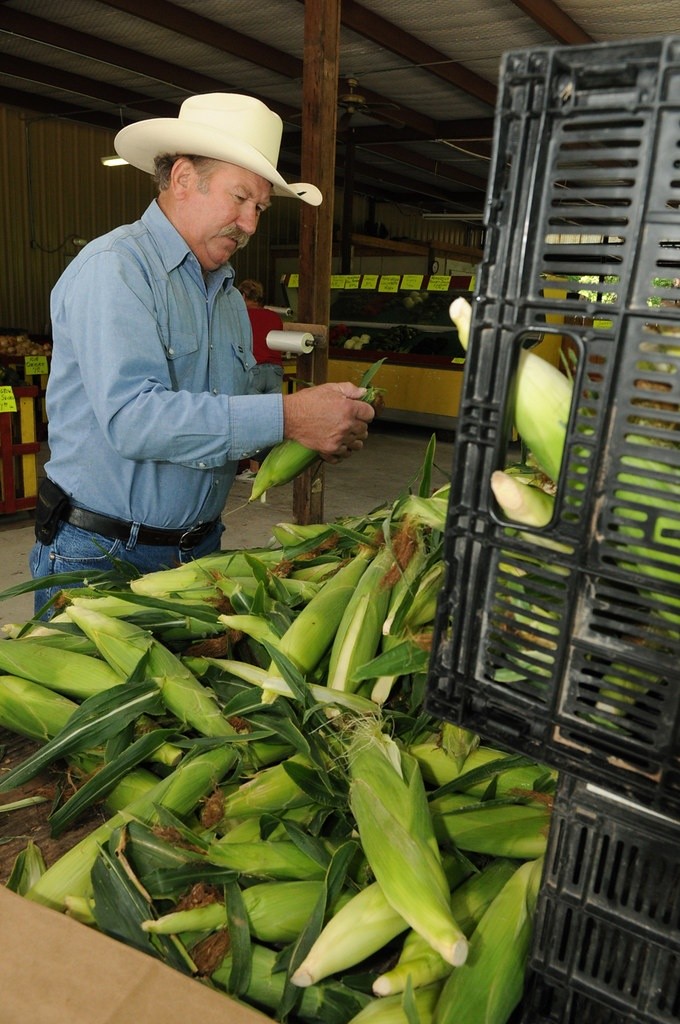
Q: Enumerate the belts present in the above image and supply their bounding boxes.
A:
[60,505,220,551]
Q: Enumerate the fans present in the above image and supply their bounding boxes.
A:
[288,67,406,131]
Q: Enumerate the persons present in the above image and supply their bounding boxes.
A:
[31,93,375,623]
[234,280,284,481]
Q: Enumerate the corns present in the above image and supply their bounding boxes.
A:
[0,296,680,1024]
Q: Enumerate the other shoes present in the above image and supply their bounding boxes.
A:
[235,468,258,483]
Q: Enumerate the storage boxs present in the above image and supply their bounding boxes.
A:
[424,37,680,1024]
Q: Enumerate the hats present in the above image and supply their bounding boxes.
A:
[114,92,325,206]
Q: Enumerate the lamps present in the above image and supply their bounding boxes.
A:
[100,108,132,168]
[421,213,484,221]
